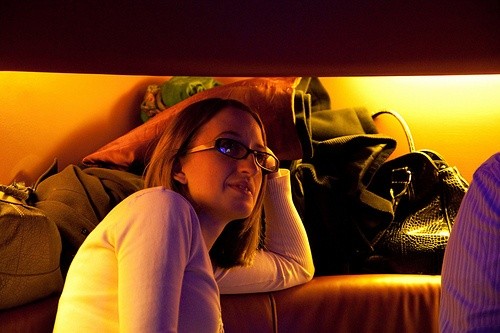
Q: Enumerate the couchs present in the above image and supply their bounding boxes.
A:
[0,273,442,333]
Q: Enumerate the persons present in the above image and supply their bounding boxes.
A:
[56,95,315,333]
[436,149,500,333]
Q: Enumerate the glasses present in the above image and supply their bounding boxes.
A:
[183,137,280,174]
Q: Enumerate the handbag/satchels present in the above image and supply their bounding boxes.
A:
[370,110,471,271]
